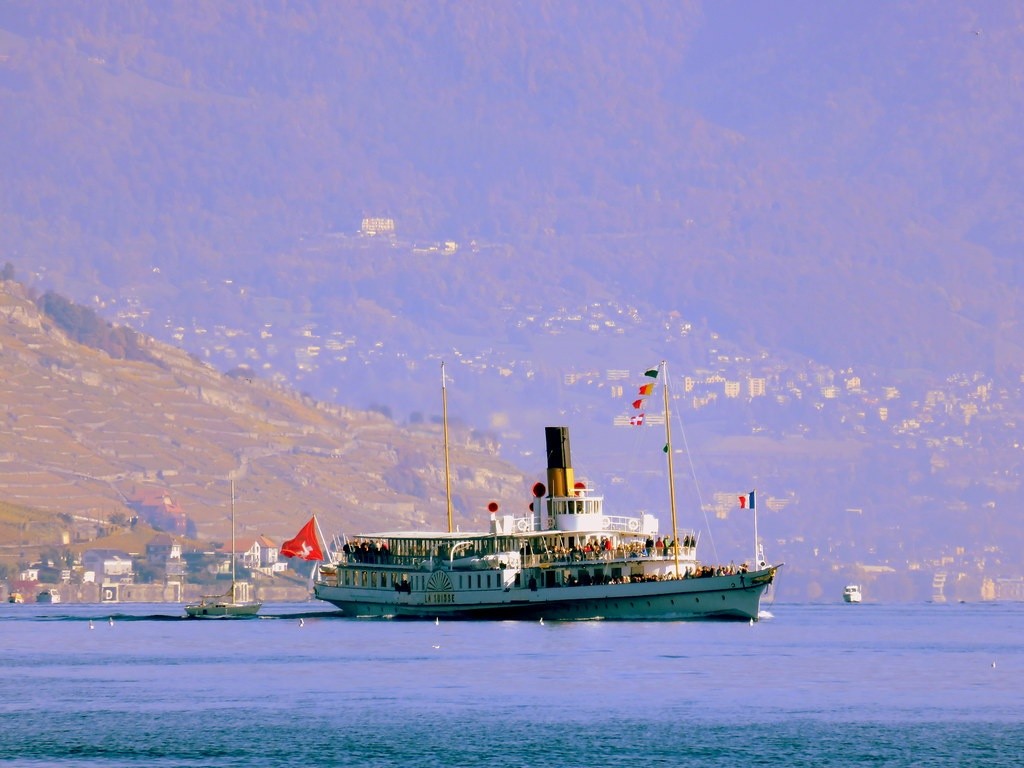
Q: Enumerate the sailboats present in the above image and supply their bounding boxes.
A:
[184,479,262,619]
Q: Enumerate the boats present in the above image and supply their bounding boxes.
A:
[8,593,22,603]
[313,359,785,622]
[843,585,862,602]
[36,589,60,604]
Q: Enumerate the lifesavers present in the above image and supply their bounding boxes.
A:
[602,517,610,528]
[517,520,527,531]
[628,519,638,531]
[548,517,555,528]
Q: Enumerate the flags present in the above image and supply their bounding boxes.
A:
[280,518,324,561]
[639,383,654,396]
[645,365,659,378]
[739,491,754,509]
[630,413,644,425]
[632,399,646,410]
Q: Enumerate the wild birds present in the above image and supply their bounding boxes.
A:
[432,645,440,648]
[991,661,996,668]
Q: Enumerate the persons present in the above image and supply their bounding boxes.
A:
[566,564,750,590]
[343,538,389,564]
[546,535,695,565]
[577,504,582,513]
